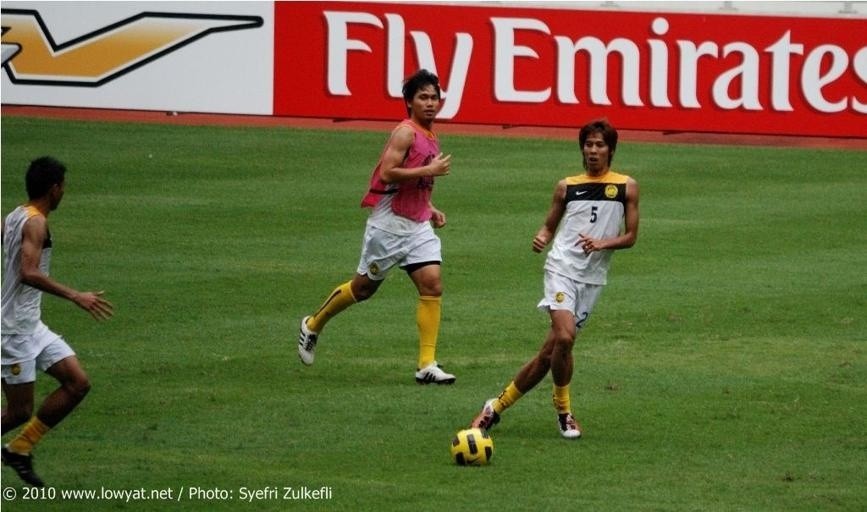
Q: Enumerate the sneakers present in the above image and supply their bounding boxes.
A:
[298,315,320,367]
[471,397,500,431]
[414,360,458,385]
[2,444,46,489]
[557,411,581,439]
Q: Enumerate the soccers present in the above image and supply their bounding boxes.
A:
[450,428,494,466]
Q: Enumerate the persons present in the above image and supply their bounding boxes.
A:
[0,155,115,489]
[296,68,458,386]
[468,118,640,439]
